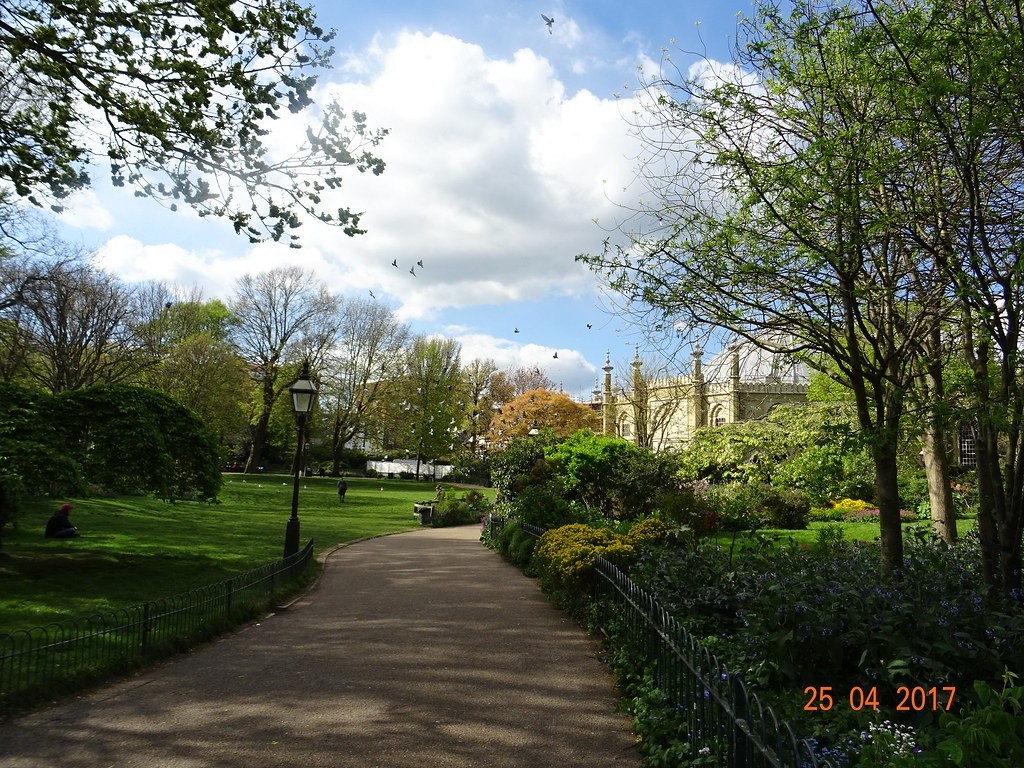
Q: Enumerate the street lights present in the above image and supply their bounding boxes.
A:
[280,355,318,561]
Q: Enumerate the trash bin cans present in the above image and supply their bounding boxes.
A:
[418,509,431,525]
[306,468,312,476]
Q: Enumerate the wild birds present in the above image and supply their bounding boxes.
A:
[541,14,554,34]
[410,266,415,276]
[515,328,519,333]
[397,352,559,461]
[587,323,592,329]
[225,453,389,492]
[418,260,423,268]
[369,290,375,298]
[553,352,557,358]
[392,259,397,267]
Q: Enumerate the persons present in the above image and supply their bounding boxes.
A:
[317,468,325,478]
[308,467,312,476]
[337,478,347,503]
[231,461,245,472]
[45,504,80,539]
[435,485,445,500]
